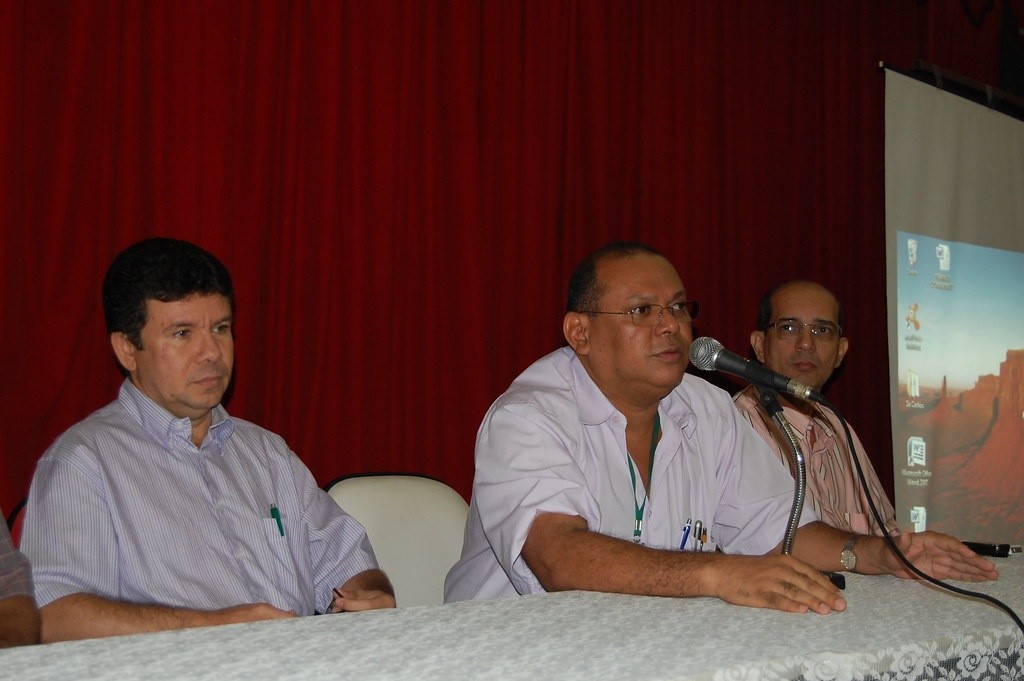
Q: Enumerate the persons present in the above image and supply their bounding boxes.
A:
[0,509,40,649]
[730,280,901,536]
[20,236,398,644]
[443,241,1000,615]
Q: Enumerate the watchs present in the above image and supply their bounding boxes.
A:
[841,534,863,573]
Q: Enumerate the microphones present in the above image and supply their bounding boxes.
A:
[689,336,833,409]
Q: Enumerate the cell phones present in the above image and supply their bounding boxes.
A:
[958,540,1010,559]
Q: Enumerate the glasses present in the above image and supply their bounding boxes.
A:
[760,315,842,340]
[575,300,700,326]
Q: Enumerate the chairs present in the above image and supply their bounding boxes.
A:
[328,471,471,608]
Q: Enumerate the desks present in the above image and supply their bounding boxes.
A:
[1,547,1024,680]
